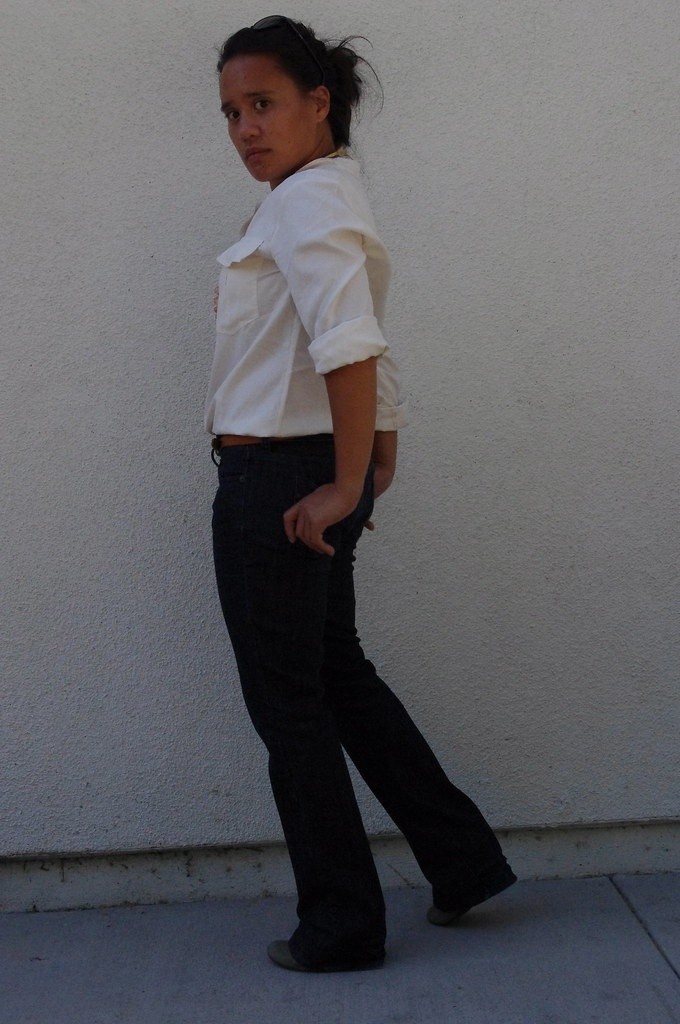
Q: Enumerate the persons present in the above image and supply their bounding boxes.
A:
[200,13,519,973]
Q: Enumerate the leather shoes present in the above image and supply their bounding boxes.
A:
[425,875,516,926]
[267,939,303,971]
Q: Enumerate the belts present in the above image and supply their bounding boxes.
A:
[215,435,263,450]
